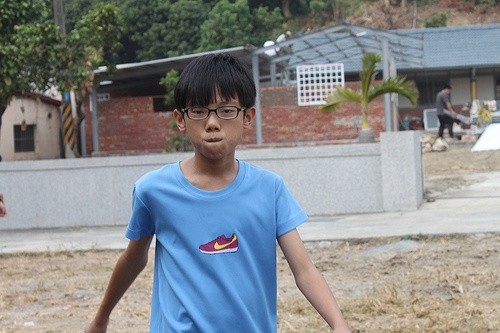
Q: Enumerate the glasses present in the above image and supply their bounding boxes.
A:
[181,106,247,121]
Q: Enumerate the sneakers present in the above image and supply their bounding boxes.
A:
[199,233,238,253]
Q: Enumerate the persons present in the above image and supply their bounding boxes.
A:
[275,30,292,56]
[436,84,458,140]
[84,53,352,333]
